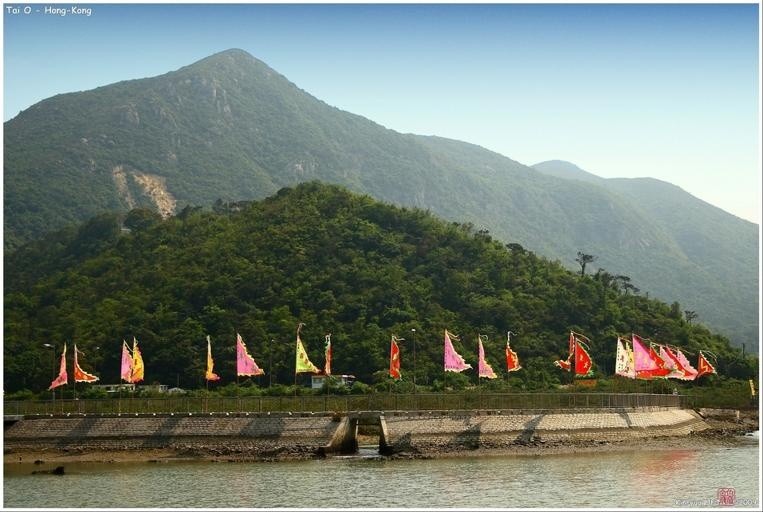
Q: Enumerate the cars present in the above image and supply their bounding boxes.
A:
[166,387,186,394]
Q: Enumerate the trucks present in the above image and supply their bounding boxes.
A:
[310,374,358,389]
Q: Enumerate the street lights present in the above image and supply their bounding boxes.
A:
[42,342,56,414]
[411,327,418,394]
[93,346,100,374]
